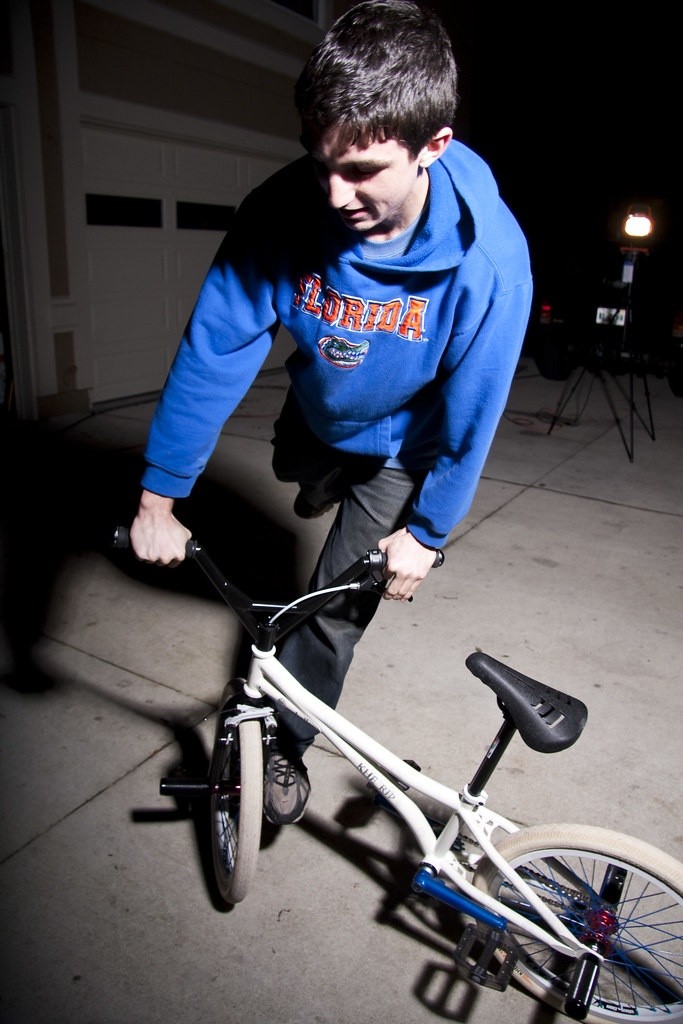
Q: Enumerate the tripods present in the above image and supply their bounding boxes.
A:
[547,247,656,464]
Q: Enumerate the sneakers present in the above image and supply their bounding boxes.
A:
[262,735,312,826]
[292,486,337,520]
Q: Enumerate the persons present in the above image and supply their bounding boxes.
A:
[129,0,533,826]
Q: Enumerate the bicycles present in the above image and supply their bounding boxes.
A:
[108,525,683,1024]
